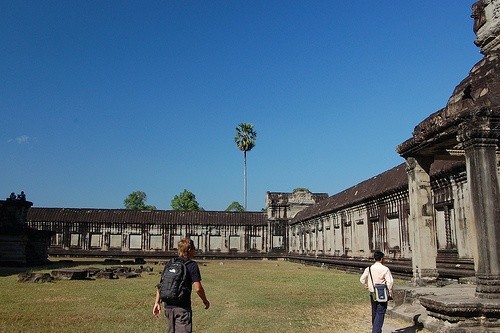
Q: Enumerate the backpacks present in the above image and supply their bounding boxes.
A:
[154,258,194,304]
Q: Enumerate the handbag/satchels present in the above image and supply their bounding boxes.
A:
[372,284,389,302]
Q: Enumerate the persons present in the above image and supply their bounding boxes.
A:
[359,250,394,332]
[152,237,210,333]
